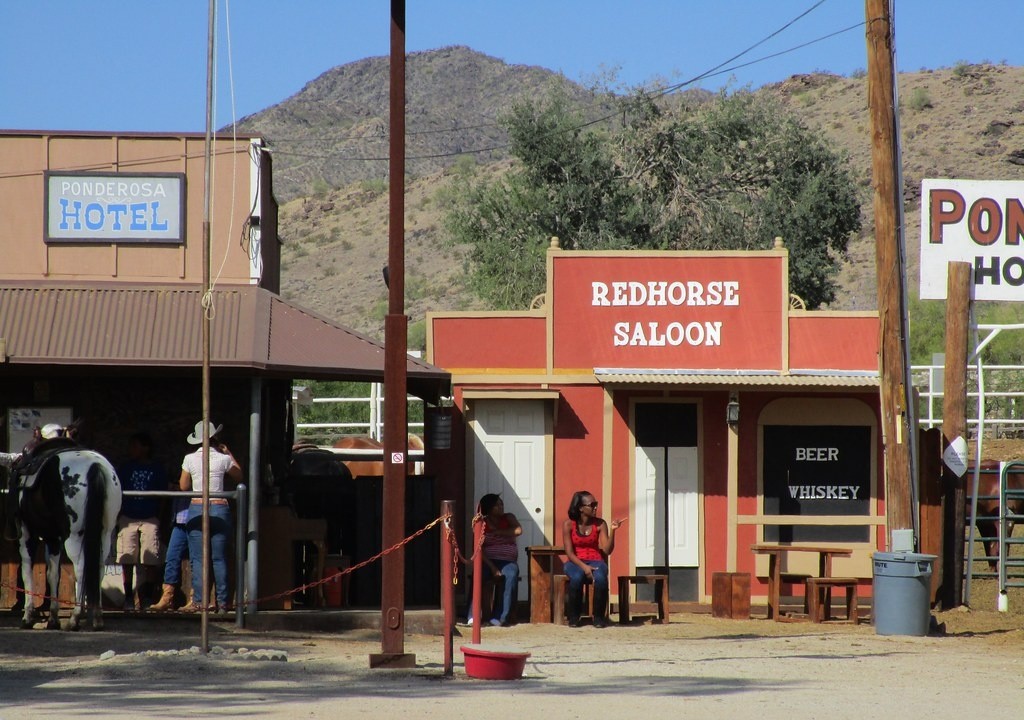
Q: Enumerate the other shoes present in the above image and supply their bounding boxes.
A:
[122,600,135,610]
[489,618,501,626]
[467,618,473,626]
[568,616,581,627]
[593,620,604,627]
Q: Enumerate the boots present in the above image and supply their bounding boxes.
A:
[150,583,175,612]
[178,589,202,613]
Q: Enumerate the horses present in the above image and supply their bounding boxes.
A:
[965,459,1024,577]
[291,431,424,480]
[0,418,124,631]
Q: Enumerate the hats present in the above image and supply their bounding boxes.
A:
[187,420,223,445]
[480,492,502,516]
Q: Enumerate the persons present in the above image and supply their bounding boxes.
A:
[563,491,628,628]
[467,494,523,626]
[150,431,201,613]
[178,420,243,613]
[117,436,174,612]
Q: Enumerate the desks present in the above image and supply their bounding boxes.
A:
[181,505,327,610]
[524,545,608,624]
[748,543,853,623]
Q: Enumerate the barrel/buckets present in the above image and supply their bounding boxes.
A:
[315,567,341,605]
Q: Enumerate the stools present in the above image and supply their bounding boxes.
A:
[807,576,858,625]
[553,575,610,625]
[617,575,669,626]
[466,574,522,622]
[711,571,752,619]
[779,573,812,584]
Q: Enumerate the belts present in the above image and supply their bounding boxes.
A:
[191,499,228,505]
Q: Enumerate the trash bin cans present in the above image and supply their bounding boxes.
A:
[870,552,938,636]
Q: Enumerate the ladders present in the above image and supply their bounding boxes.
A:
[1000,461,1024,591]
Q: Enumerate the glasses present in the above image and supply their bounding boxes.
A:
[584,501,598,507]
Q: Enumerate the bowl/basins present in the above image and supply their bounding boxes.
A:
[462,646,531,679]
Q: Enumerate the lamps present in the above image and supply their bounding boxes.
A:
[429,413,453,455]
[726,394,740,427]
